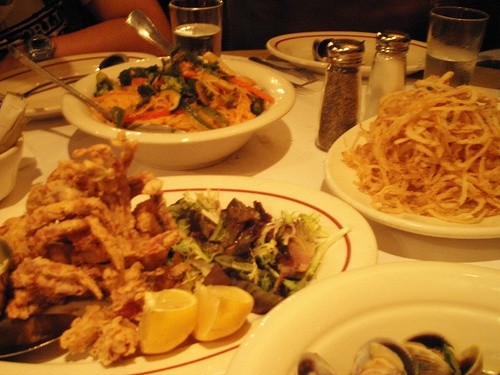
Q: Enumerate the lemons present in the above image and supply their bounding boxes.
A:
[138,288,199,354]
[190,284,254,342]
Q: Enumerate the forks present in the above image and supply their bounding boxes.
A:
[249,56,316,80]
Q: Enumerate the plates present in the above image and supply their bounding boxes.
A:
[0,174,377,375]
[225,260,500,375]
[324,84,500,239]
[266,31,428,80]
[0,52,158,121]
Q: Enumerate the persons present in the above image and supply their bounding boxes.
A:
[0,0,173,73]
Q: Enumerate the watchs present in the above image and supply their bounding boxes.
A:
[27,33,56,62]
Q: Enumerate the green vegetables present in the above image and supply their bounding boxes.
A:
[164,190,350,314]
[92,47,263,132]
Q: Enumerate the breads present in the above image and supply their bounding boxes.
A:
[0,93,27,154]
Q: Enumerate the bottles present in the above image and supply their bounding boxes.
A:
[315,38,365,152]
[364,31,411,119]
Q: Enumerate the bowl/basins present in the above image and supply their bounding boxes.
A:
[60,55,297,171]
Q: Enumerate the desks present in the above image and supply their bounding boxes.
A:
[0,50,500,375]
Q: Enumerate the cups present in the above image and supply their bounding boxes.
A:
[423,5,489,87]
[168,0,223,57]
